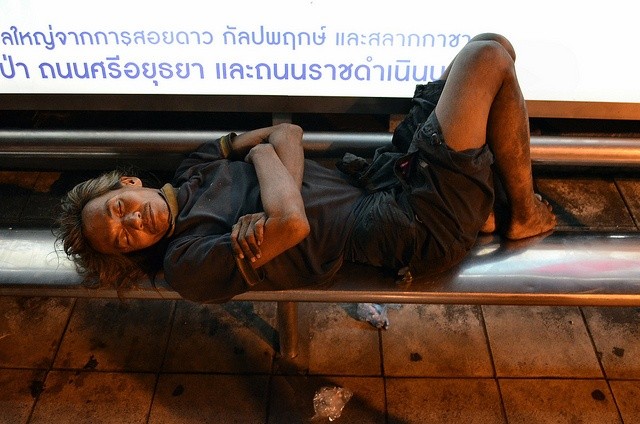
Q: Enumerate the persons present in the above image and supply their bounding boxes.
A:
[52,33,558,303]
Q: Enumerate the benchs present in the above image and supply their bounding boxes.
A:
[1,115,640,309]
